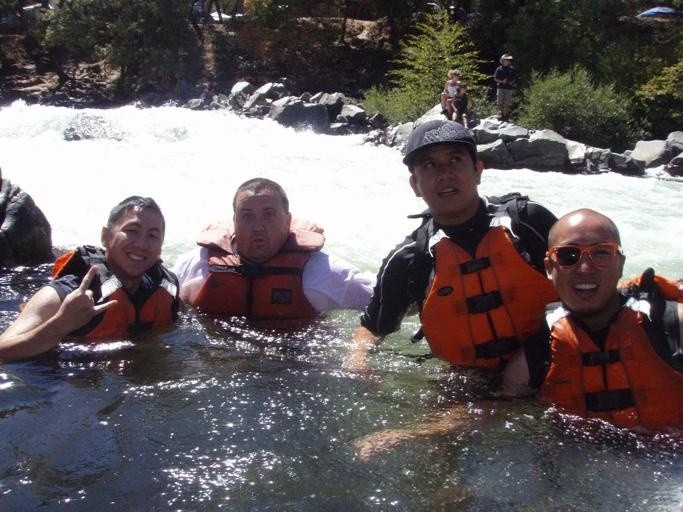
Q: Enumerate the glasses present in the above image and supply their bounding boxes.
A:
[546,244,626,268]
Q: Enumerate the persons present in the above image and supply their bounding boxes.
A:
[180,178,377,328]
[351,208,683,463]
[0,195,178,365]
[341,119,558,376]
[441,52,517,130]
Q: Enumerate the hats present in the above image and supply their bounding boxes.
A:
[403,119,477,168]
